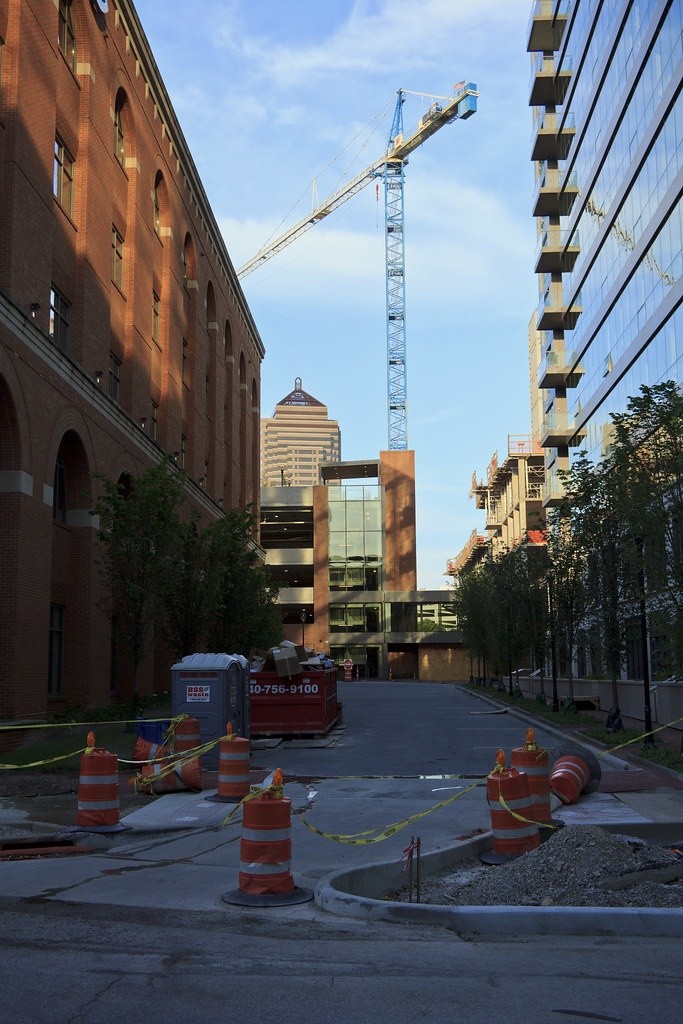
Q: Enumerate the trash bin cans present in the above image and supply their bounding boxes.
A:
[136,717,170,746]
[250,667,339,736]
[170,653,251,771]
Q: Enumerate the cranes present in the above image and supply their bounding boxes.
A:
[237,81,479,451]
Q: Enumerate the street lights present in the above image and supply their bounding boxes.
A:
[633,523,657,749]
[545,559,557,714]
[300,609,306,648]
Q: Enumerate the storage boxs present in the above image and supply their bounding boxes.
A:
[135,717,170,746]
[249,637,339,736]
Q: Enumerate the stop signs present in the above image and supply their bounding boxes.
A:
[344,659,353,670]
